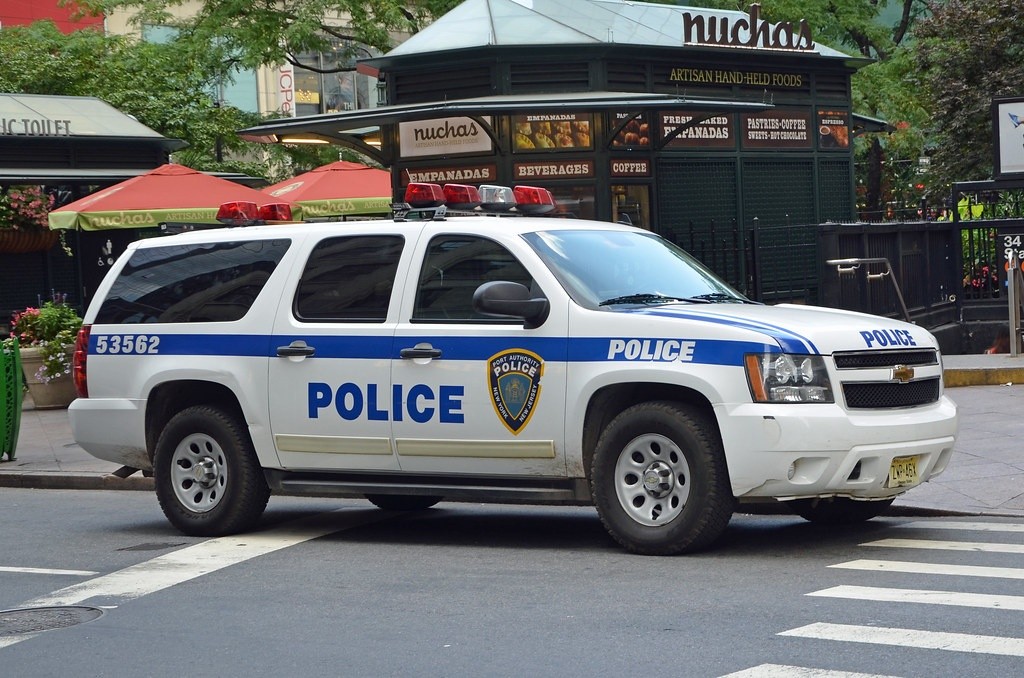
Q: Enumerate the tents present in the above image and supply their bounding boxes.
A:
[48,160,304,317]
[260,161,393,222]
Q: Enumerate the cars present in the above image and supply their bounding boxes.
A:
[67,180,960,556]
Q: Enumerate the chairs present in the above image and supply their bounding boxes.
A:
[444,268,485,320]
[413,267,449,320]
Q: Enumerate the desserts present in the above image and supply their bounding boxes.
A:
[517,121,589,149]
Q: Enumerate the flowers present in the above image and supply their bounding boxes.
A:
[8,288,83,386]
[0,185,73,258]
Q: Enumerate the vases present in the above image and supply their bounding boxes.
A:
[0,226,61,254]
[16,343,83,410]
